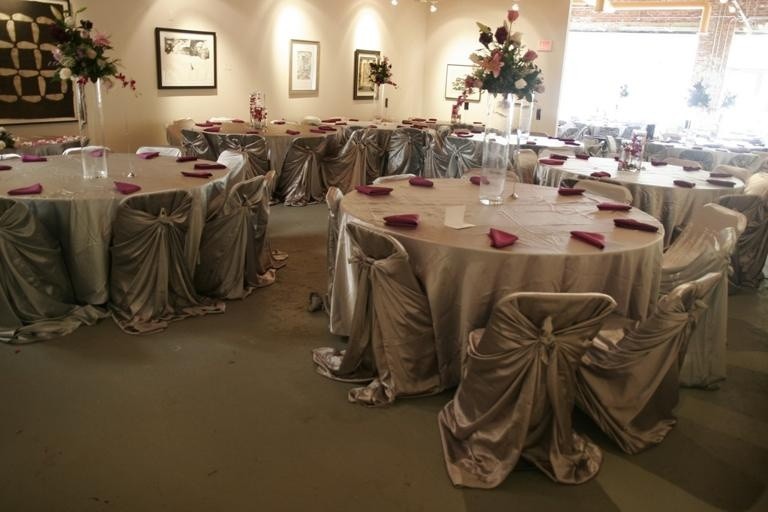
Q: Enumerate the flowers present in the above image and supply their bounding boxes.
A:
[42,4,145,124]
[368,56,398,100]
[451,10,545,120]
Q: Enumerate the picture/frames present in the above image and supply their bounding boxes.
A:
[155,27,217,89]
[354,49,380,101]
[289,38,320,98]
[445,64,481,103]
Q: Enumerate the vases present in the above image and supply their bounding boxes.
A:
[478,92,514,206]
[517,91,535,140]
[373,83,385,125]
[74,76,109,180]
